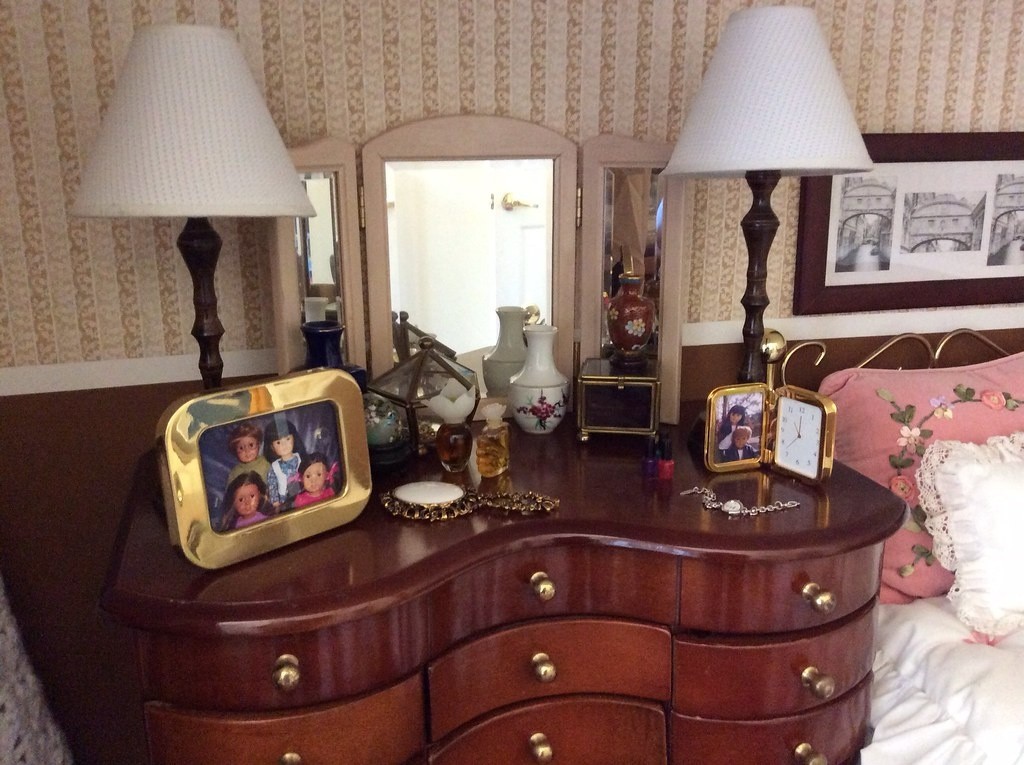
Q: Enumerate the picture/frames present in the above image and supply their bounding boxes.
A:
[150,363,375,574]
[791,130,1024,316]
[703,382,771,474]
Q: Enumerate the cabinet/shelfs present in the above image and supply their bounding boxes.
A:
[99,422,914,764]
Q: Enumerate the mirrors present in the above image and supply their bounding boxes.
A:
[580,133,687,358]
[271,136,368,384]
[359,114,579,424]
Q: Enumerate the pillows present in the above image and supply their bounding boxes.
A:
[917,431,1023,641]
[814,351,1024,607]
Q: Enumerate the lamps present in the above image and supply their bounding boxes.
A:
[657,5,877,447]
[65,20,322,391]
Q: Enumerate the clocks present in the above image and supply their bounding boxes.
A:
[770,385,837,486]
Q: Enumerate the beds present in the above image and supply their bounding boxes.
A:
[677,328,1024,764]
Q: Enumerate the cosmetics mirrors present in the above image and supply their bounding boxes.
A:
[373,479,563,524]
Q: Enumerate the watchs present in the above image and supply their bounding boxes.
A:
[675,484,801,518]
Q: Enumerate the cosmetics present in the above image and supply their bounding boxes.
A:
[473,402,511,478]
[420,375,478,474]
[638,436,658,480]
[656,439,674,482]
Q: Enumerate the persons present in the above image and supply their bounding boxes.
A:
[724,425,761,464]
[717,403,748,463]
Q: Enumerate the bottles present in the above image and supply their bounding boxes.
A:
[288,318,366,397]
[475,403,510,479]
